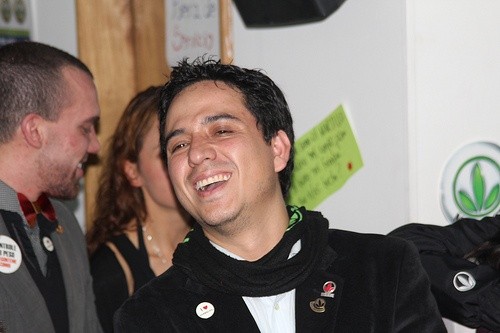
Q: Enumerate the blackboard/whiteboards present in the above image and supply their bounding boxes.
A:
[164,0,233,66]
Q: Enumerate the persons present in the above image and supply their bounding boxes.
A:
[84,82,194,331]
[386,214,500,333]
[0,41,101,333]
[109,53,448,333]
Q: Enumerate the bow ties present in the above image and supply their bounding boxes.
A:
[17,192,56,229]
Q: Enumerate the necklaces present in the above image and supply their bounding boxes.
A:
[142,224,174,271]
